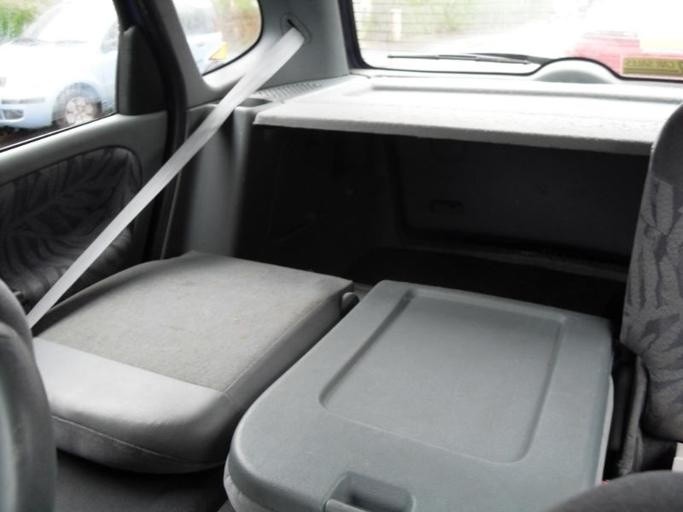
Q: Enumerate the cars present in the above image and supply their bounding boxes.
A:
[0,0,225,130]
